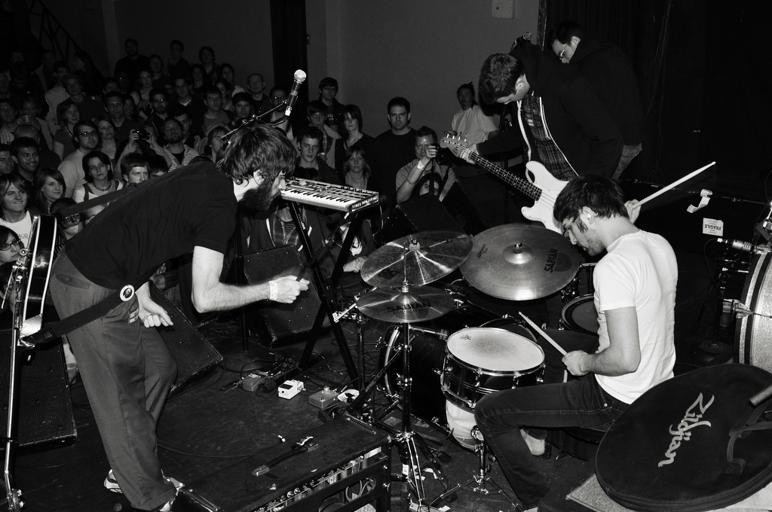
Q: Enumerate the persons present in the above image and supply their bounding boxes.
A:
[545,16,647,188]
[1,25,507,359]
[470,178,681,511]
[49,122,311,511]
[481,37,625,199]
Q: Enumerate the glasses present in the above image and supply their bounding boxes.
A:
[564,216,578,239]
[272,95,286,101]
[559,44,568,61]
[77,132,97,137]
[0,238,21,251]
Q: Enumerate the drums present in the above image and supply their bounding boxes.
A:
[384,304,538,452]
[735,239,772,371]
[559,262,601,336]
[441,328,546,410]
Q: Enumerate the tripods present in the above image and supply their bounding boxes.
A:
[391,326,452,507]
[425,439,524,512]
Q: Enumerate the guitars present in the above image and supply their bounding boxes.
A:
[439,129,570,235]
[0,213,59,510]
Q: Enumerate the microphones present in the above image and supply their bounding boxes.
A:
[284,70,306,118]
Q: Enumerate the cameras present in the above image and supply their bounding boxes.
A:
[133,130,149,139]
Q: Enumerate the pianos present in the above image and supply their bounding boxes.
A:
[280,174,380,211]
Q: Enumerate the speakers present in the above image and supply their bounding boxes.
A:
[173,413,392,512]
[149,280,223,403]
[0,325,78,451]
[241,245,331,349]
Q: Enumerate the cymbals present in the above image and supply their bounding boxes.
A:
[461,222,578,301]
[363,230,473,289]
[355,285,454,324]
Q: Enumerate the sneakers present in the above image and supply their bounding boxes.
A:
[104,469,186,497]
[520,427,547,457]
